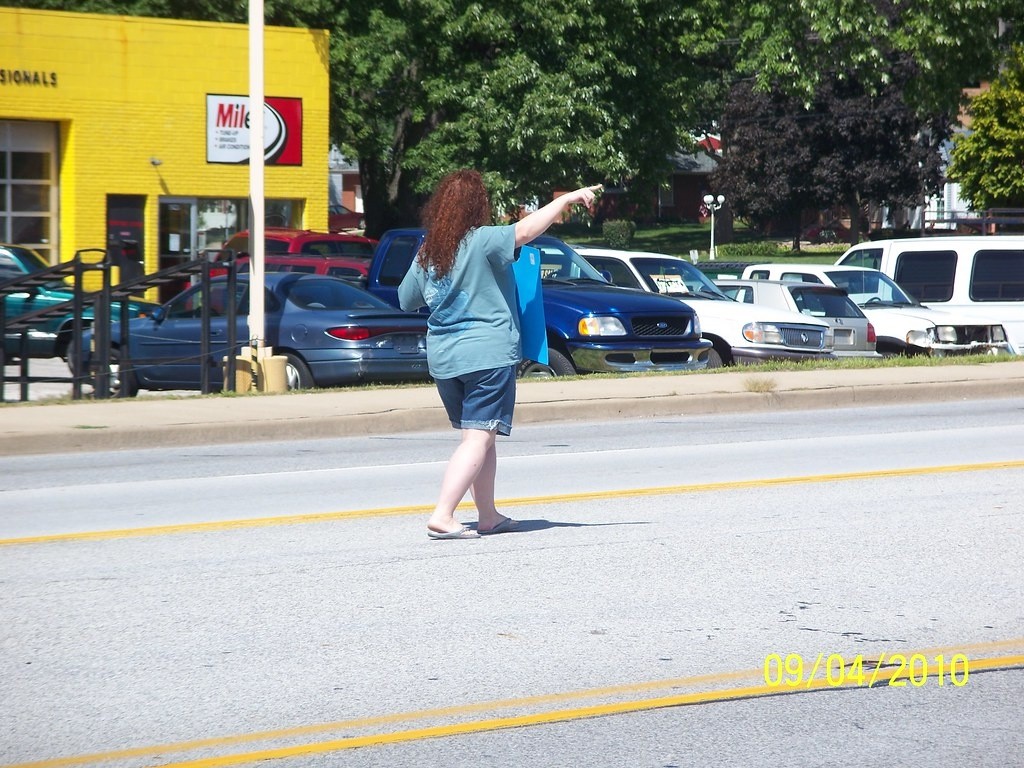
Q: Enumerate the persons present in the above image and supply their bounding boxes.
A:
[397,169,603,539]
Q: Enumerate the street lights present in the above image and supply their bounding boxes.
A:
[704,194,726,261]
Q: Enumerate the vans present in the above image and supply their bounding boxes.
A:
[835,232,1024,356]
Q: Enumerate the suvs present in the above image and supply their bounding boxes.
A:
[185,251,371,316]
[683,279,882,359]
[539,246,838,370]
[0,243,164,385]
[368,229,713,382]
[214,227,376,268]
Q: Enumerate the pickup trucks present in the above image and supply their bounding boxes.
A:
[734,261,1018,357]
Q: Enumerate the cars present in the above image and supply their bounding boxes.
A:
[66,270,433,399]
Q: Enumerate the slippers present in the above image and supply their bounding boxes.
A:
[477,518,520,534]
[428,526,481,539]
